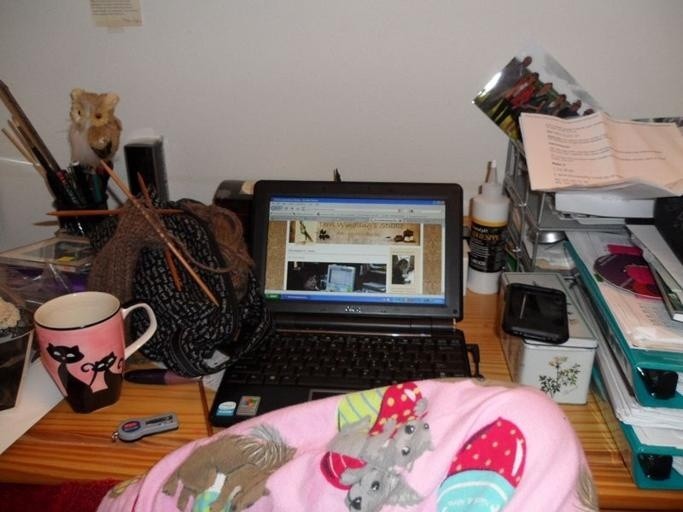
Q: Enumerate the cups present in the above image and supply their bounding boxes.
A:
[31,289,159,416]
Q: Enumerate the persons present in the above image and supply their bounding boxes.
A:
[480,55,594,143]
[294,269,318,290]
[392,259,412,285]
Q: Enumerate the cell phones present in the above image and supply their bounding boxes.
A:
[502,282,569,344]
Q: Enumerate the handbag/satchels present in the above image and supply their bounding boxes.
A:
[90,197,265,378]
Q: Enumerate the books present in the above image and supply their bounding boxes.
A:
[629,232,683,323]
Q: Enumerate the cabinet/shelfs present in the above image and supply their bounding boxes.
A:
[502,139,626,279]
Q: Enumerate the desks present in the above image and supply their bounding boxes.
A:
[0,291,683,512]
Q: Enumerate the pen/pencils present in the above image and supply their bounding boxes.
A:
[0,82,111,238]
[23,262,71,309]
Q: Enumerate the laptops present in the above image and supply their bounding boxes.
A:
[208,181,472,428]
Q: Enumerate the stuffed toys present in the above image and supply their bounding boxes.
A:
[65,87,123,170]
